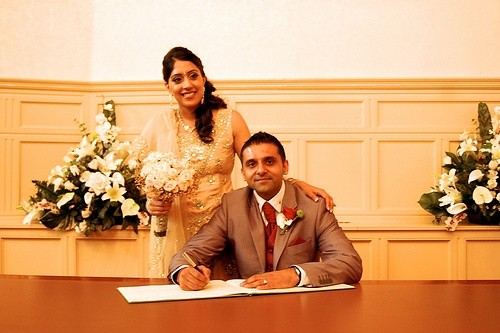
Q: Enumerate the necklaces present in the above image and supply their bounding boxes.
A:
[176,109,195,133]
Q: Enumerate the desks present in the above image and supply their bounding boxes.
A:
[0,274,500,333]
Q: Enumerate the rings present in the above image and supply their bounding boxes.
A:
[264,279,267,283]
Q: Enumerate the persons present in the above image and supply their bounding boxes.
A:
[135,46,337,278]
[167,131,363,291]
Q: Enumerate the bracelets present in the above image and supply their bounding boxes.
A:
[291,266,301,285]
[284,176,299,184]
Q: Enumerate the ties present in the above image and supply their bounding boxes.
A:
[262,201,277,272]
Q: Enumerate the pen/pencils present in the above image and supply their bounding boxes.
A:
[181,252,203,274]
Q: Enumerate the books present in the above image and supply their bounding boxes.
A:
[117,275,355,304]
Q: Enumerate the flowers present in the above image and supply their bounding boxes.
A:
[277,207,303,235]
[418,102,500,231]
[137,150,193,237]
[17,101,152,238]
